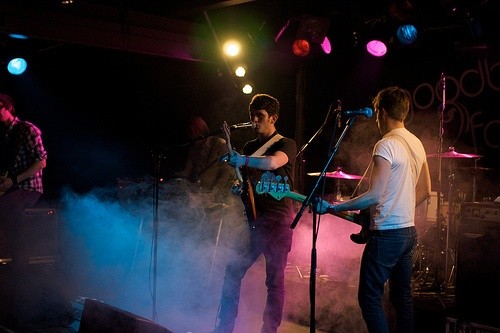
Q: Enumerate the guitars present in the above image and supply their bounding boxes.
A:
[223,122,257,230]
[255,172,372,245]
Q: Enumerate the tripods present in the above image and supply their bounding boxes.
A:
[405,74,456,295]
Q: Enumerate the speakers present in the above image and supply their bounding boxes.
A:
[454,219,500,333]
[0,207,58,262]
[380,280,455,333]
[79,299,172,333]
[0,259,75,329]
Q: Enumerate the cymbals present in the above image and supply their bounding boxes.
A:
[427,151,482,159]
[307,171,366,180]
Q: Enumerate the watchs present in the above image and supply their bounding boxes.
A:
[329,204,336,213]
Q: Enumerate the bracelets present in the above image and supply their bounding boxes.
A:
[10,176,18,186]
[244,155,249,167]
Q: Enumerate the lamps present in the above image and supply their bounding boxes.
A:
[291,6,419,57]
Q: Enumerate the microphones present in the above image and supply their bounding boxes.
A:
[345,107,372,117]
[231,121,256,129]
[337,100,341,128]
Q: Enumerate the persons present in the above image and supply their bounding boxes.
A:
[176,115,236,213]
[216,93,297,333]
[0,92,48,285]
[313,86,432,333]
